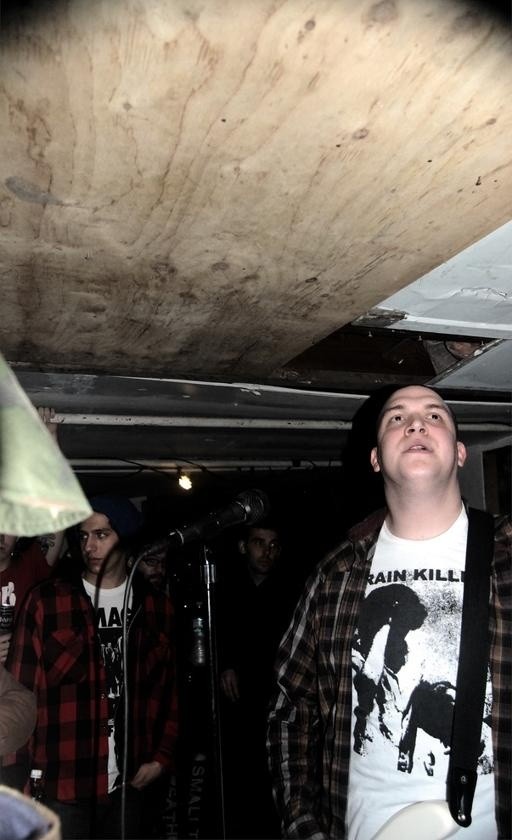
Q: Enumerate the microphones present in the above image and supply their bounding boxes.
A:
[148,492,265,556]
[191,597,207,666]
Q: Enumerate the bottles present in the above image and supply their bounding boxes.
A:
[27,769,49,806]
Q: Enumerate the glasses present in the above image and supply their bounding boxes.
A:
[142,556,172,569]
[246,538,280,554]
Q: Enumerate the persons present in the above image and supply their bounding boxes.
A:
[2,407,65,666]
[266,384,511,840]
[1,662,41,760]
[1,497,182,840]
[211,518,301,839]
[126,531,182,839]
